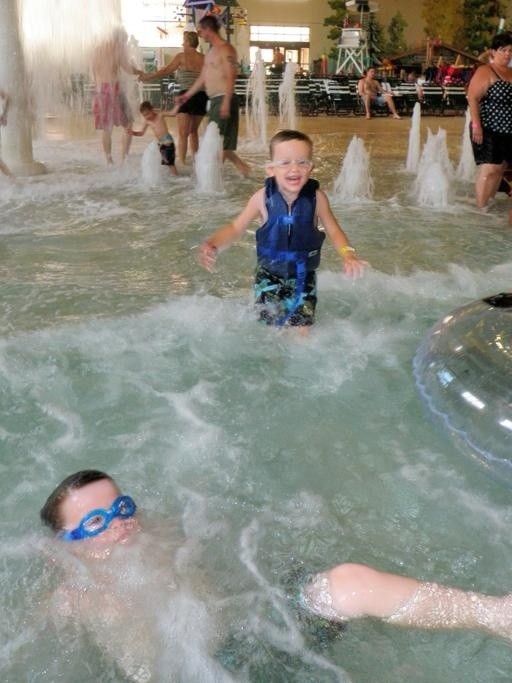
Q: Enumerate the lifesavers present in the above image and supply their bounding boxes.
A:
[411,293,511,488]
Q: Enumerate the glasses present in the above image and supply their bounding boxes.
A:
[272,158,310,171]
[64,495,137,541]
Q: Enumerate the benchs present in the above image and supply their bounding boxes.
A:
[68,78,468,116]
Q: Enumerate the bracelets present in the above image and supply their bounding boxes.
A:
[338,246,356,256]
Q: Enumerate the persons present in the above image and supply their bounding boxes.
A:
[36,467,512,682]
[198,128,367,339]
[467,32,512,208]
[89,13,251,179]
[239,48,466,119]
